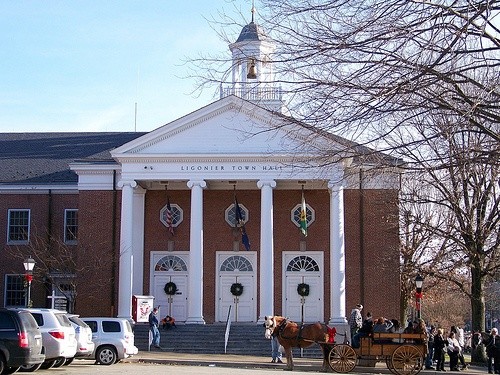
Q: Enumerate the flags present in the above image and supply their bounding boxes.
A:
[234,194,251,252]
[165,194,176,237]
[300,192,309,238]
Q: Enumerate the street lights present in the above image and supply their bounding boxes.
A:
[23,255,37,308]
[414,273,423,318]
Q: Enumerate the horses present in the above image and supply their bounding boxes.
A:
[263,315,336,373]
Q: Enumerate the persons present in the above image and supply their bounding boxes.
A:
[161,314,177,330]
[270,330,285,364]
[485,327,500,374]
[349,303,363,348]
[351,311,373,350]
[374,316,491,372]
[148,307,161,349]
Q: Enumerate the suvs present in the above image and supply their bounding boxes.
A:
[64,313,95,366]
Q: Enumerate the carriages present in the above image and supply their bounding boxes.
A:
[264,315,425,375]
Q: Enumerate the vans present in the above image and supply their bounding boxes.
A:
[80,318,138,365]
[16,308,77,372]
[0,308,45,375]
